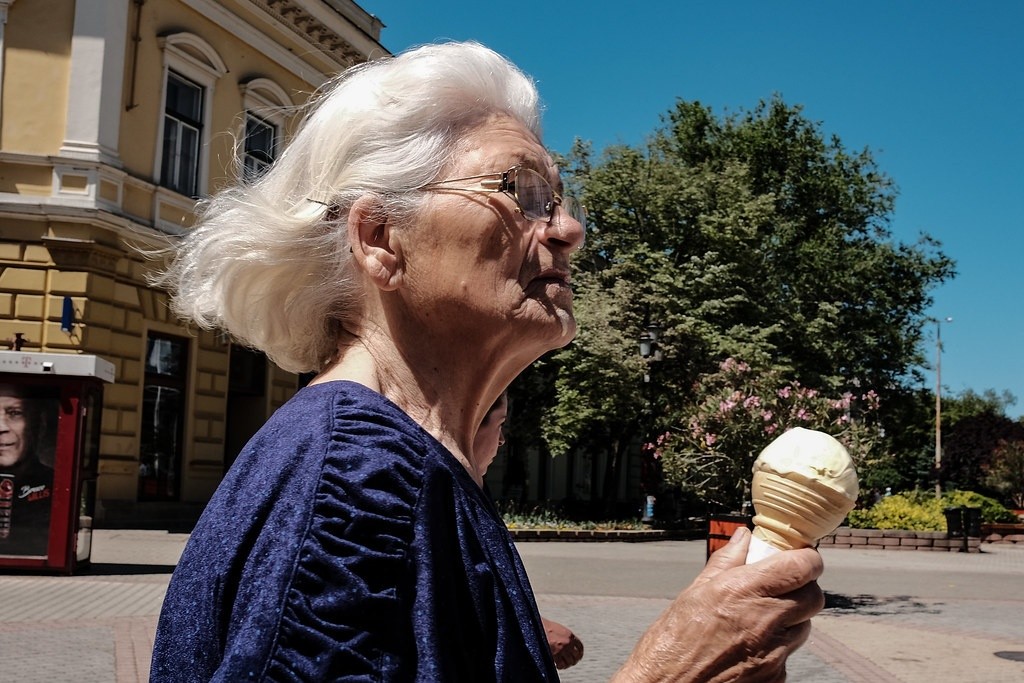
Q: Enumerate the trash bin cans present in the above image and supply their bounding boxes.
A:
[942,505,982,538]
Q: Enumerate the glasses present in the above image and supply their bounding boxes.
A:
[393,167,587,250]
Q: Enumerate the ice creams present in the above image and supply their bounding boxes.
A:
[744,428,859,566]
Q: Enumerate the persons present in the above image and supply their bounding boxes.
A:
[0,373,55,556]
[121,41,824,683]
[473,390,584,670]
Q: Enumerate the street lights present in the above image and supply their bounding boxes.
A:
[922,315,953,500]
